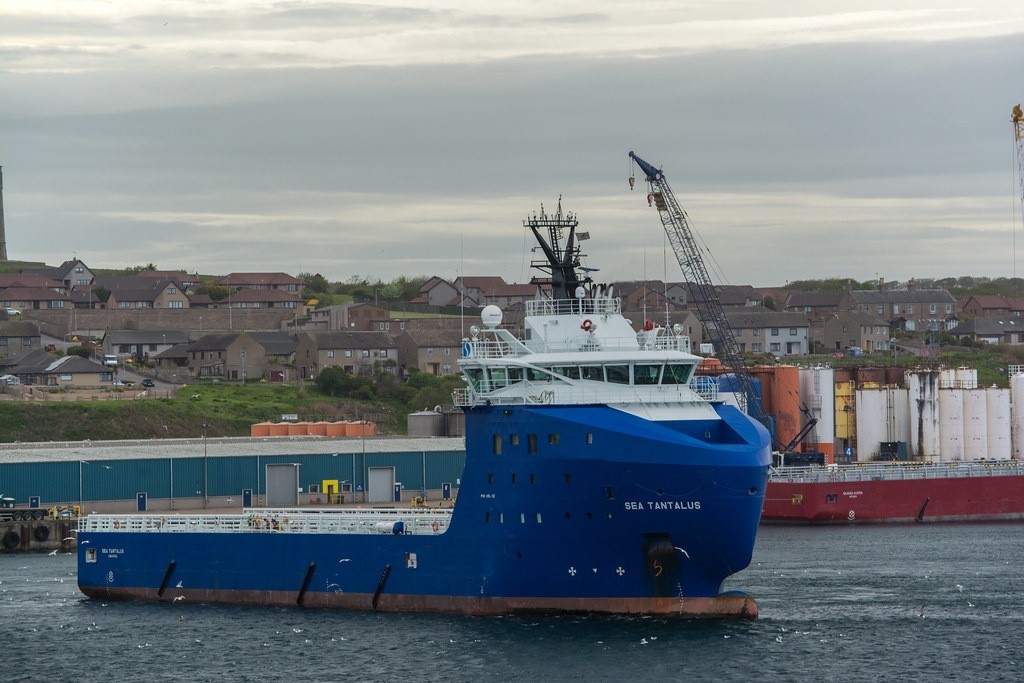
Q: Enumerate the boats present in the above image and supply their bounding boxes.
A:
[76,193,773,625]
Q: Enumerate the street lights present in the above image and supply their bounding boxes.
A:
[893,327,900,367]
[80,459,90,515]
[375,288,380,307]
[163,334,166,369]
[229,286,234,329]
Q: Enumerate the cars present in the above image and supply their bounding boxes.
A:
[113,379,124,386]
[5,307,21,317]
[141,378,154,387]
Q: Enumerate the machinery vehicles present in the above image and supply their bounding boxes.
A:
[628,150,825,476]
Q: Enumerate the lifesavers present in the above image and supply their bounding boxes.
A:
[829,473,835,479]
[433,523,438,532]
[115,521,120,529]
[4,532,19,548]
[35,526,49,541]
